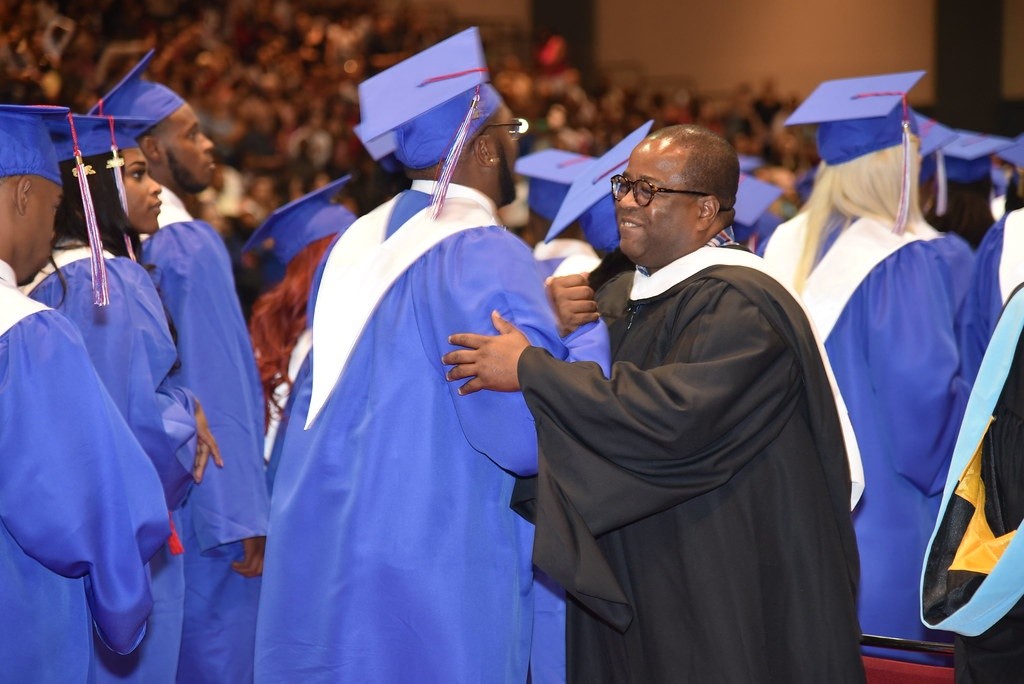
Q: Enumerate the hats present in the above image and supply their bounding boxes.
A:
[50,114,156,262]
[352,26,500,221]
[83,48,184,144]
[511,71,1024,236]
[244,174,360,281]
[0,103,109,307]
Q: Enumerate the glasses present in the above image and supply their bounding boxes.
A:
[610,174,709,207]
[467,117,530,147]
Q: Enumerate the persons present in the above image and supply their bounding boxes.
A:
[0,0,1024,684]
